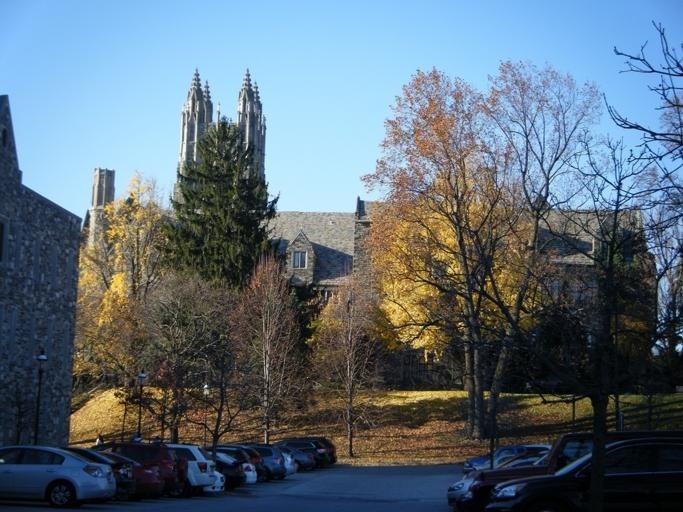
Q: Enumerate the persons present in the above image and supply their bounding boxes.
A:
[133,433,145,443]
[151,435,162,443]
[95,434,105,446]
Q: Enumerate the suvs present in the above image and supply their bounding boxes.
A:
[89,441,189,497]
[165,442,220,494]
[276,436,338,467]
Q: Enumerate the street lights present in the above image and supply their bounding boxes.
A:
[33,347,47,445]
[138,367,146,438]
[203,382,210,447]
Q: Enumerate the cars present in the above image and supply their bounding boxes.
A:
[0,445,116,510]
[448,431,683,512]
[58,447,138,502]
[204,438,316,491]
[95,451,164,498]
